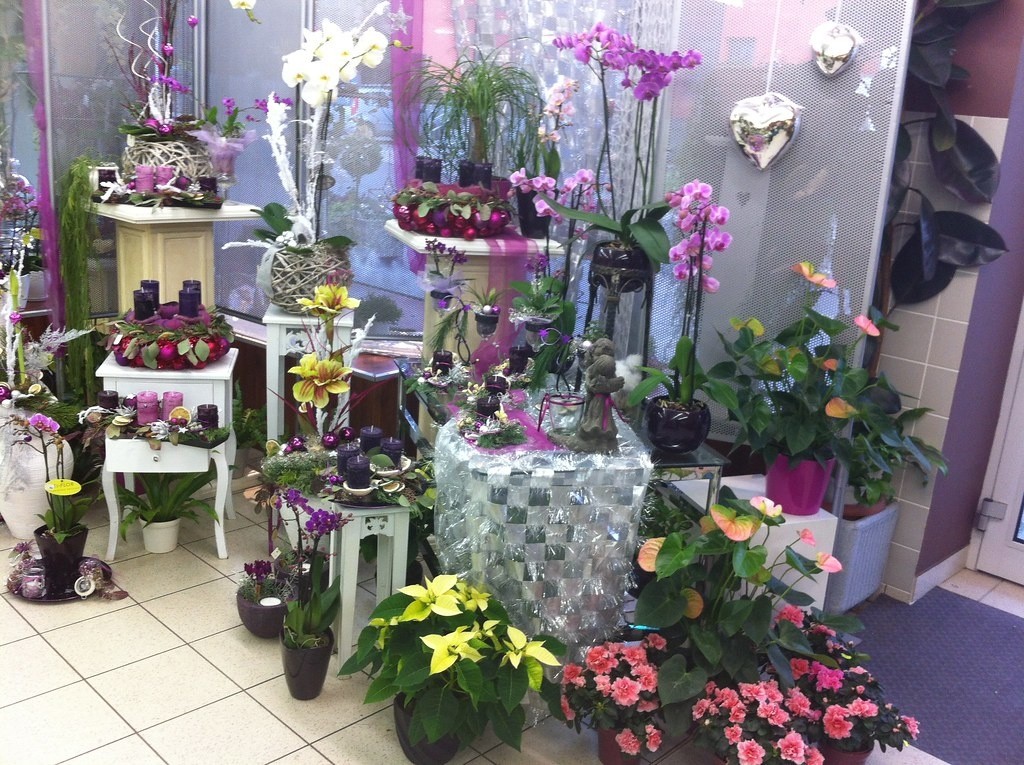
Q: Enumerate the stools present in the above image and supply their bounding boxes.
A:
[328,500,410,678]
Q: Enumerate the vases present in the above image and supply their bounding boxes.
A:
[470,113,488,164]
[822,732,875,765]
[595,239,650,294]
[645,397,713,455]
[4,273,31,312]
[0,426,75,540]
[768,444,836,519]
[33,522,88,600]
[517,187,561,241]
[27,268,51,302]
[235,483,460,765]
[596,719,642,765]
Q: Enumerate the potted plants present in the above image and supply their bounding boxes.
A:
[394,241,548,335]
[116,471,221,555]
[821,0,1007,619]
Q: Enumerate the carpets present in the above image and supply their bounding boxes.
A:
[845,586,1024,765]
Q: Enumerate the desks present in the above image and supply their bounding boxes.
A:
[260,297,354,444]
[80,200,263,522]
[384,218,565,462]
[94,346,240,520]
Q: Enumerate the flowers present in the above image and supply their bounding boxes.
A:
[421,236,468,278]
[333,572,923,765]
[507,19,919,765]
[0,0,418,562]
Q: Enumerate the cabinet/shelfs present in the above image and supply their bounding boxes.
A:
[667,471,840,641]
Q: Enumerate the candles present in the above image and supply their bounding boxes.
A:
[97,168,220,196]
[431,344,533,418]
[197,403,218,430]
[98,391,182,427]
[134,279,202,320]
[337,425,402,489]
